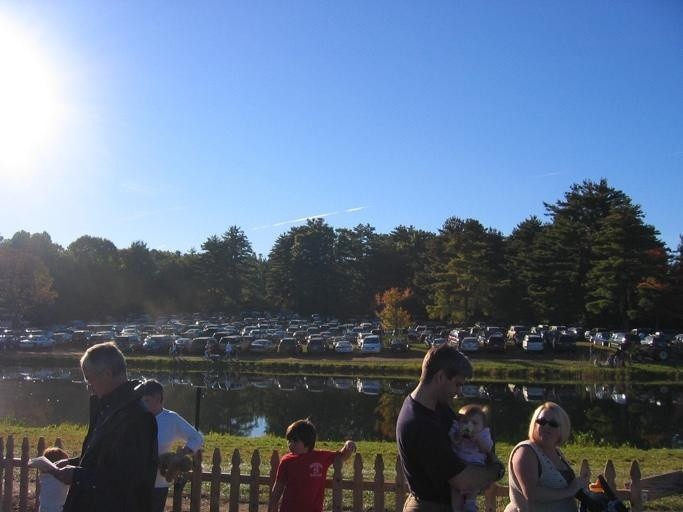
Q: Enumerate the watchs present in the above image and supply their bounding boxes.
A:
[495,461,505,481]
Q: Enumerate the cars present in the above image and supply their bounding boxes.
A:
[1,313,406,356]
[408,321,683,363]
[136,372,683,408]
[1,368,83,383]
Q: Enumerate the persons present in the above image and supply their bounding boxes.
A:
[169,371,241,399]
[134,379,203,512]
[395,343,504,512]
[50,342,159,512]
[168,339,241,361]
[504,402,588,512]
[38,448,70,512]
[448,405,494,512]
[269,420,357,512]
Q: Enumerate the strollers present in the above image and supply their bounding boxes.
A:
[568,474,630,512]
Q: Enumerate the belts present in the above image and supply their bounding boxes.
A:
[412,494,450,505]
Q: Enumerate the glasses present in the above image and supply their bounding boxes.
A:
[536,419,559,427]
